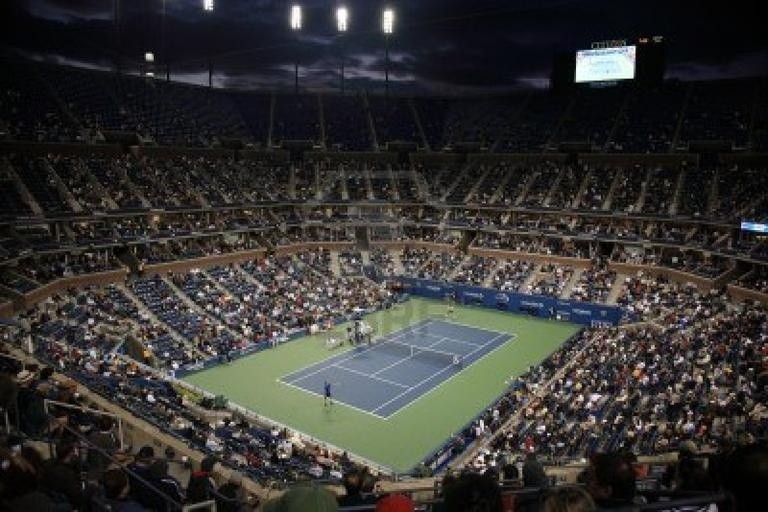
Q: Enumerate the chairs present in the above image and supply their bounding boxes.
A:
[0,57,767,512]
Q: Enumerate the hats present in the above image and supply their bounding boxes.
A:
[261,486,337,511]
[376,494,415,512]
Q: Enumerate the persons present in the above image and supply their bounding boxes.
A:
[2,50,768,512]
